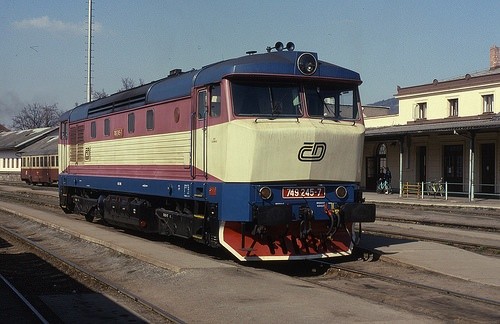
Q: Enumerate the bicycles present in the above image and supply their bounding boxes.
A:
[376,178,392,195]
[427,177,446,199]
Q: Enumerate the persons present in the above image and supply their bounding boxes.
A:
[376,166,394,195]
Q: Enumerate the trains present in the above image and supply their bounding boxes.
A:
[21,149,58,186]
[58,41,377,262]
